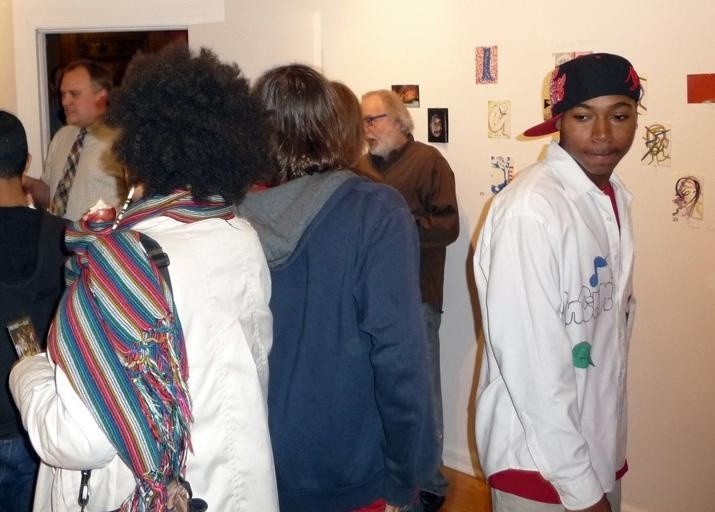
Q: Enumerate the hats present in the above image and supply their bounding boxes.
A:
[523,52,639,137]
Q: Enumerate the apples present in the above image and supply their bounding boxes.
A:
[82,200,116,228]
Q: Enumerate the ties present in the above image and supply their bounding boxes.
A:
[50,127,88,213]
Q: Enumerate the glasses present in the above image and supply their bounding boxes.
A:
[365,114,388,125]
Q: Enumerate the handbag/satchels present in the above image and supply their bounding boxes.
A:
[111,496,206,512]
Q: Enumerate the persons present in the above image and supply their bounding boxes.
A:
[474,52,640,510]
[1,44,459,512]
